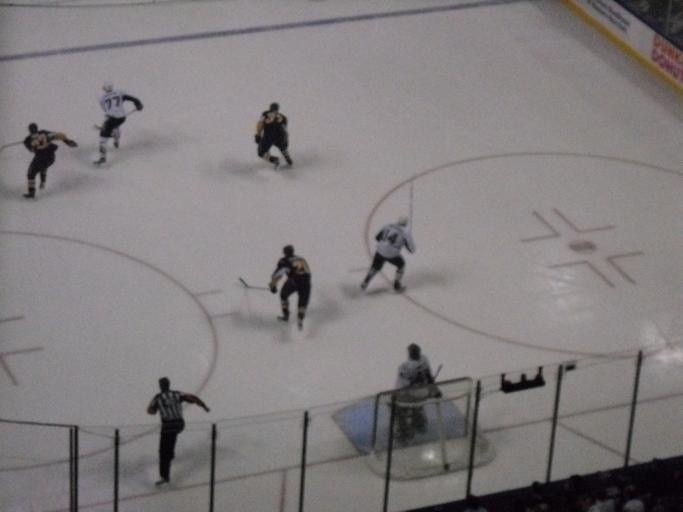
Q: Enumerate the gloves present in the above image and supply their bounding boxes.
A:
[255,135,262,144]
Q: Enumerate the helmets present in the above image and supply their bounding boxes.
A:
[102,83,113,91]
[399,214,409,225]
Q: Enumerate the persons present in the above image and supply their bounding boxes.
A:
[253,101,294,170]
[20,123,72,198]
[414,450,682,512]
[267,245,311,328]
[391,342,440,439]
[357,216,415,293]
[89,82,143,167]
[146,376,209,488]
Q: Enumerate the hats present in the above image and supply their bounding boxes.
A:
[407,344,420,355]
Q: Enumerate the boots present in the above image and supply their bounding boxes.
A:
[297,313,304,324]
[40,178,45,186]
[394,280,401,289]
[361,279,370,288]
[154,478,169,487]
[93,154,107,164]
[281,311,289,321]
[23,192,35,198]
[284,155,292,165]
[113,140,119,147]
[268,156,279,166]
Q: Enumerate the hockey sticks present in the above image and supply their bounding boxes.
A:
[239,278,270,292]
